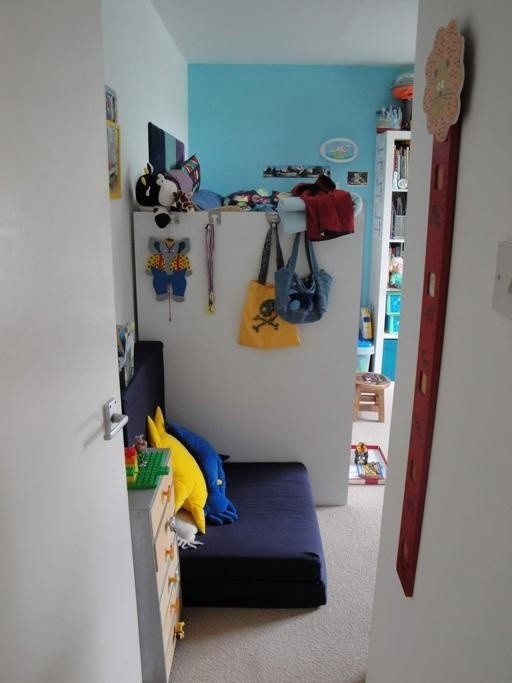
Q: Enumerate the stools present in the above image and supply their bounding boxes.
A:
[353,373,391,422]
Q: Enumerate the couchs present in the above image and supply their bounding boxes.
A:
[119,340,328,609]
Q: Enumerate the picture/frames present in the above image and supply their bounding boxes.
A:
[107,121,122,200]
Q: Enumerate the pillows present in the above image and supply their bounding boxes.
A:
[174,508,204,550]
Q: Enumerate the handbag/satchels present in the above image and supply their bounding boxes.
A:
[238,224,301,350]
[275,231,332,324]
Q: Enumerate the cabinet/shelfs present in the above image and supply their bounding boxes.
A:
[369,130,411,381]
[128,448,181,683]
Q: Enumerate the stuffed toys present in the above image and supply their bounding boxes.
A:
[167,419,237,526]
[147,405,207,533]
[135,163,177,227]
